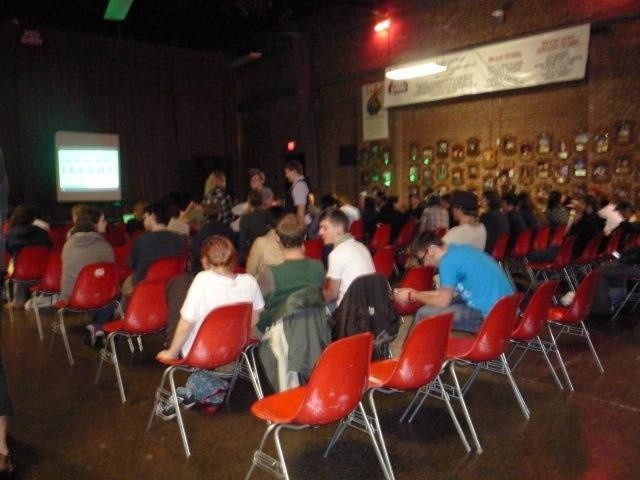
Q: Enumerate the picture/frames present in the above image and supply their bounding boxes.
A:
[360,119,639,212]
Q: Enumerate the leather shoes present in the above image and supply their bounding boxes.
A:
[94,330,106,350]
[83,325,94,345]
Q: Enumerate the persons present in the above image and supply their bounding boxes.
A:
[1,201,51,307]
[60,189,516,362]
[479,190,638,254]
[281,160,310,219]
[1,357,13,478]
[247,171,272,212]
[200,171,234,225]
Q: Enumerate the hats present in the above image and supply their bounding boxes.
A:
[444,190,480,209]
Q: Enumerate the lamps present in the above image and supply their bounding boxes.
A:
[364,2,394,38]
[381,0,449,83]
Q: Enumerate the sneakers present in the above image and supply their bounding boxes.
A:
[175,387,196,408]
[152,401,176,420]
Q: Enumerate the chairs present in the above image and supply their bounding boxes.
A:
[408,286,531,427]
[334,309,472,455]
[2,217,448,356]
[486,219,639,325]
[512,267,609,392]
[502,278,567,413]
[94,278,167,407]
[145,301,263,459]
[239,328,390,479]
[52,261,134,368]
